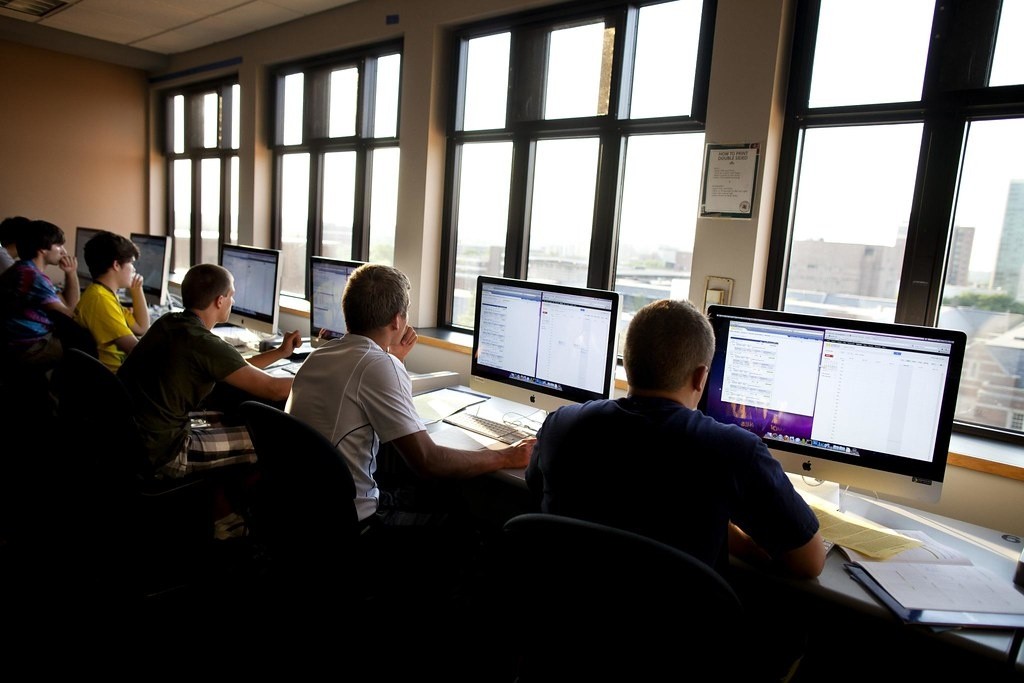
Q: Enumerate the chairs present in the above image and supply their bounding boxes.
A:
[0,305,748,683]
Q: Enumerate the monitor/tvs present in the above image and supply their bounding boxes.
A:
[75,227,103,282]
[219,243,284,343]
[309,256,371,348]
[125,232,173,311]
[698,304,968,507]
[471,275,623,414]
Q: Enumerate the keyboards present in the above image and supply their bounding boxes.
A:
[282,362,303,374]
[443,412,535,443]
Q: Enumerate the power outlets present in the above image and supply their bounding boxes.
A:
[705,277,733,306]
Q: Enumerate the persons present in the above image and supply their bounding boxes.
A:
[0,216,32,275]
[0,220,81,340]
[115,263,303,540]
[72,231,151,373]
[523,298,826,682]
[284,265,537,566]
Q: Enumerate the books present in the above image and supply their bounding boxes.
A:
[835,530,1024,631]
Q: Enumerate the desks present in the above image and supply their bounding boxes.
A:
[72,268,1024,665]
